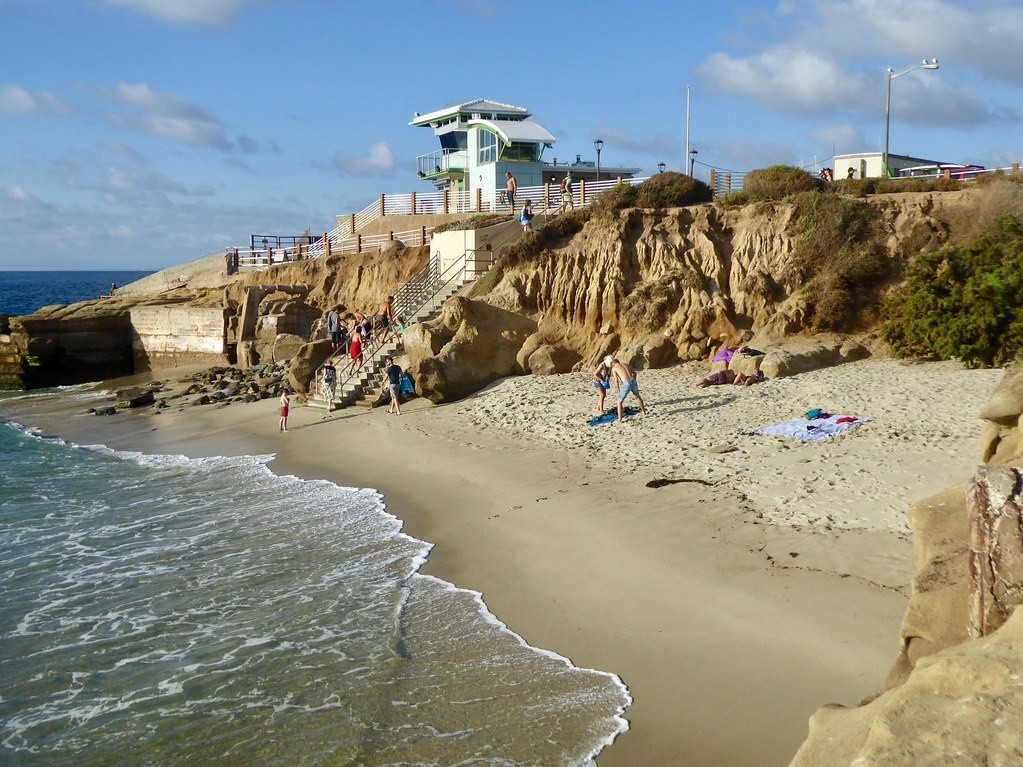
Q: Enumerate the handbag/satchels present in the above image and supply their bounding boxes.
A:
[522,206,535,222]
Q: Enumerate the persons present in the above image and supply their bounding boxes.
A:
[522,200,534,231]
[349,326,365,376]
[733,368,762,385]
[344,312,371,348]
[824,170,832,182]
[376,296,394,343]
[279,388,290,432]
[321,359,336,412]
[848,168,854,179]
[396,317,405,338]
[505,172,517,216]
[593,355,614,414]
[611,358,646,423]
[561,171,573,214]
[381,357,403,416]
[697,369,737,388]
[328,307,343,356]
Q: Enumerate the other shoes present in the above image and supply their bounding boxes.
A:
[507,214,514,216]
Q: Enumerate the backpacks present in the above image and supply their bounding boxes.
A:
[560,177,570,193]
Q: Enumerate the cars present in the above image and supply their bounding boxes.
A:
[939,169,982,184]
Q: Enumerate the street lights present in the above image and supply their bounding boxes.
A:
[884,64,940,180]
[551,176,556,205]
[689,149,698,179]
[594,139,603,194]
